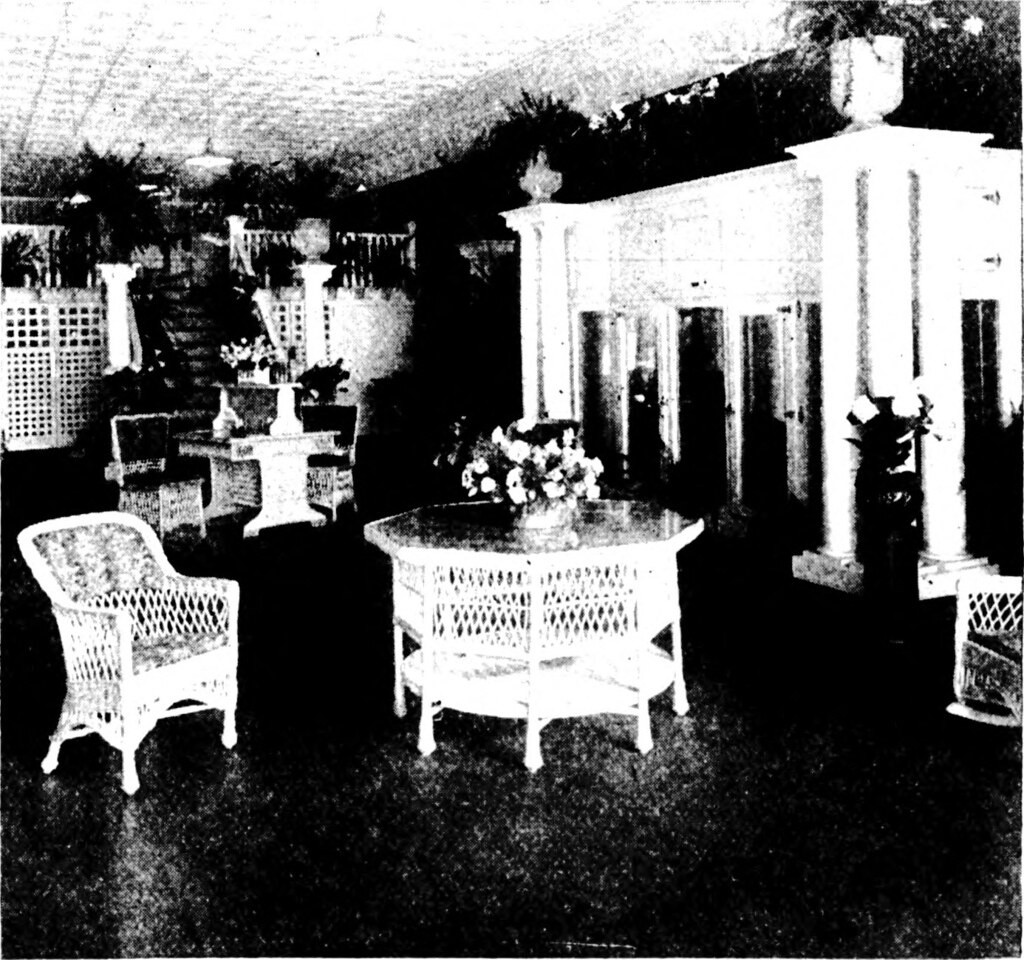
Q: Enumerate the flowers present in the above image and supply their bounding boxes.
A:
[219,334,287,375]
[462,417,605,504]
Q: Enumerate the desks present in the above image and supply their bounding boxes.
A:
[362,500,706,774]
[210,380,306,437]
[960,629,1023,727]
[177,426,342,537]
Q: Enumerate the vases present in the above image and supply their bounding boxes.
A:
[514,492,581,527]
[236,366,275,384]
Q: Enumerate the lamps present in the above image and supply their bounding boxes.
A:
[183,134,235,168]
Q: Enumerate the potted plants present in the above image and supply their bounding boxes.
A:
[484,88,599,206]
[257,148,362,257]
[771,0,954,137]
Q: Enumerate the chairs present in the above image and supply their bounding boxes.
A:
[105,413,210,540]
[303,405,362,521]
[17,511,240,793]
[946,574,1023,728]
